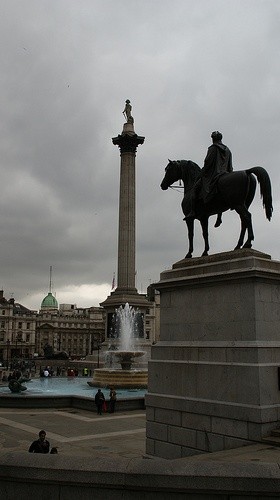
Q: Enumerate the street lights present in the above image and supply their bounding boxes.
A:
[6,338,12,371]
[96,345,102,369]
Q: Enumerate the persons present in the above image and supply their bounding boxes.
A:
[15,363,25,372]
[109,387,116,413]
[201,131,233,227]
[57,366,66,376]
[50,447,57,454]
[83,367,89,376]
[40,366,54,376]
[67,367,78,376]
[122,99,133,123]
[2,369,21,381]
[95,389,105,415]
[33,363,37,373]
[26,371,30,378]
[29,430,49,453]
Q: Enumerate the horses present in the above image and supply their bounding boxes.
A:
[159,158,276,260]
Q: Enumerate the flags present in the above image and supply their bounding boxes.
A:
[112,276,115,289]
